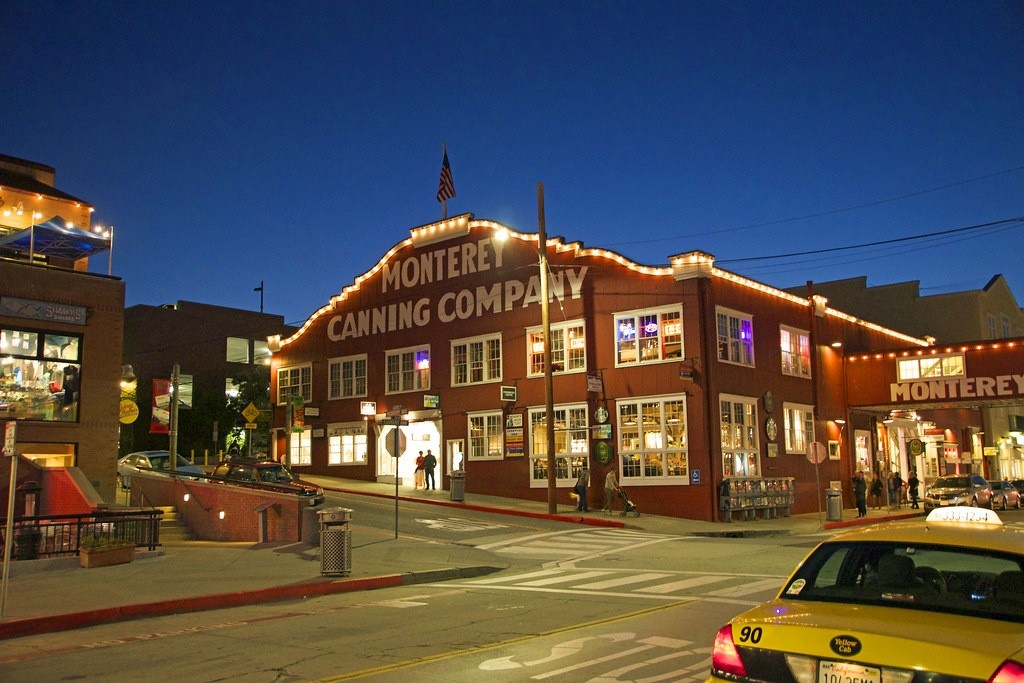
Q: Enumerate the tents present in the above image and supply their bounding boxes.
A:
[0,215,113,276]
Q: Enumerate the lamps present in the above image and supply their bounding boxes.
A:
[667,415,678,422]
[882,416,893,423]
[122,364,135,377]
[624,418,636,425]
[835,415,845,423]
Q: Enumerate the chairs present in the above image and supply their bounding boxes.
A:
[996,570,1024,606]
[878,554,917,588]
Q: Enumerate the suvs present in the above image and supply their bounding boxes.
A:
[923,472,995,514]
[207,458,325,508]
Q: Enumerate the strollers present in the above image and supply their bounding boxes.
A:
[617,488,640,518]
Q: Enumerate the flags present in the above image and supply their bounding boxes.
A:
[436,149,455,202]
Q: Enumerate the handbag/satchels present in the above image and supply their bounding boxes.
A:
[415,468,418,473]
[574,486,579,495]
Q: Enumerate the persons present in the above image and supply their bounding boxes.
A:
[887,471,920,509]
[229,440,247,454]
[870,472,882,509]
[537,460,542,468]
[601,464,622,512]
[855,471,866,519]
[574,470,592,512]
[414,449,437,490]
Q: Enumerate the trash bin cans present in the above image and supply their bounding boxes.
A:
[824,488,844,520]
[449,470,467,502]
[316,507,354,575]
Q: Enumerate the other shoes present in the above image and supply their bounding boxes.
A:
[425,488,429,490]
[910,505,914,509]
[433,487,436,490]
[915,506,919,509]
[577,507,591,512]
[601,508,617,516]
[856,516,866,519]
[414,487,417,489]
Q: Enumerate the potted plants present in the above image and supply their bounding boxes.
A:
[78,535,136,568]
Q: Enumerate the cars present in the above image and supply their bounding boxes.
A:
[115,450,211,490]
[1009,480,1024,506]
[985,480,1021,512]
[705,505,1024,683]
